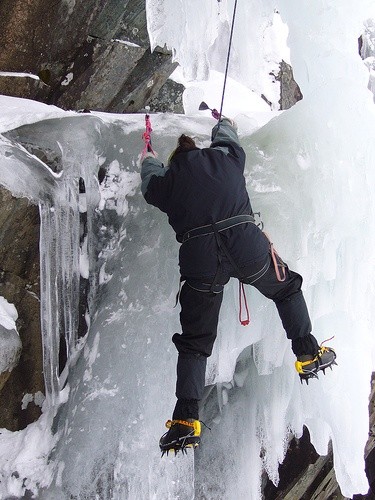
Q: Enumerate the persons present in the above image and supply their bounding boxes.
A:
[140,117,336,449]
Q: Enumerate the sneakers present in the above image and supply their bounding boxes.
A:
[158,419,203,458]
[295,345,336,385]
